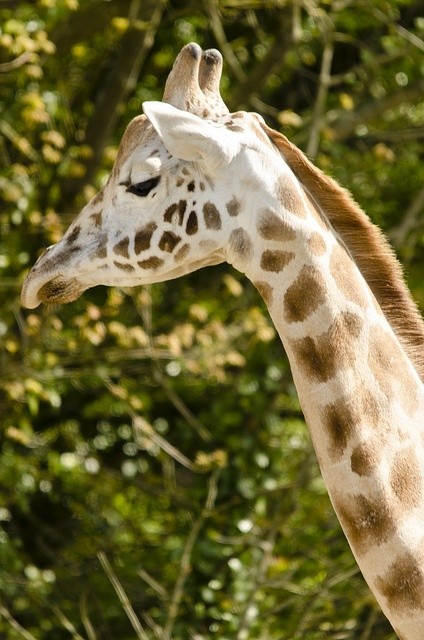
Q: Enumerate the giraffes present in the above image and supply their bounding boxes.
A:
[19,43,424,640]
